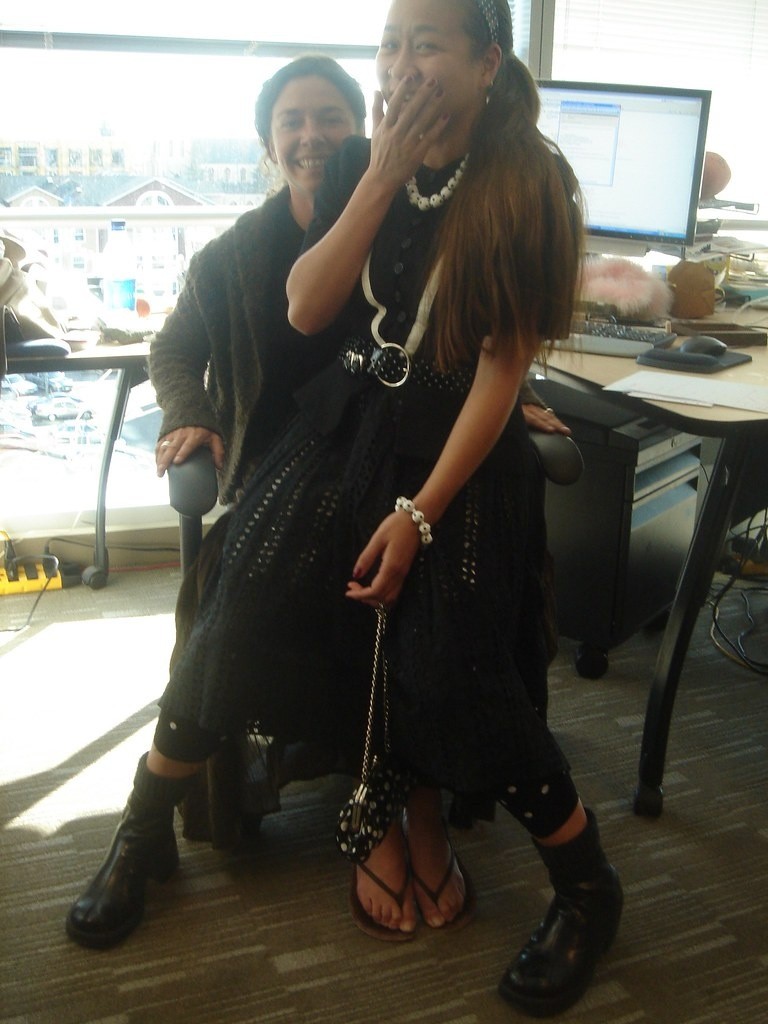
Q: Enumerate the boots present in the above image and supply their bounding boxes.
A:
[498,807,623,1017]
[66,750,190,949]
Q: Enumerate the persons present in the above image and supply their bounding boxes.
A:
[64,0,629,1011]
[150,55,572,938]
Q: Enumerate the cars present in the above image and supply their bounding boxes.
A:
[0,382,16,400]
[35,397,92,421]
[1,373,38,395]
[25,370,74,392]
[26,392,84,414]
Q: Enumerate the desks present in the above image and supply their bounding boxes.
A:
[537,306,768,819]
[0,341,150,589]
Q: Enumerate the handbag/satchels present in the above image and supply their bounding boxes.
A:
[336,744,406,864]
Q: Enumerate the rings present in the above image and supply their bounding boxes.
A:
[545,408,553,413]
[162,441,170,445]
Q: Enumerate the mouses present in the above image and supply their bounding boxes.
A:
[680,336,727,358]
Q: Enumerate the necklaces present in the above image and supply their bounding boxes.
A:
[403,153,471,210]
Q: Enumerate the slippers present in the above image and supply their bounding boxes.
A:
[403,811,478,934]
[348,858,420,943]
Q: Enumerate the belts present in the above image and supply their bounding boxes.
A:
[338,334,477,394]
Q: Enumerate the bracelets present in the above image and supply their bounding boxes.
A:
[394,496,434,546]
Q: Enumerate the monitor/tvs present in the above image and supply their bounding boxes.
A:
[531,79,713,259]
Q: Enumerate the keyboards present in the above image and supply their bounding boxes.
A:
[541,318,677,358]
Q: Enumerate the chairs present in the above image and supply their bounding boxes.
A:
[166,420,584,848]
